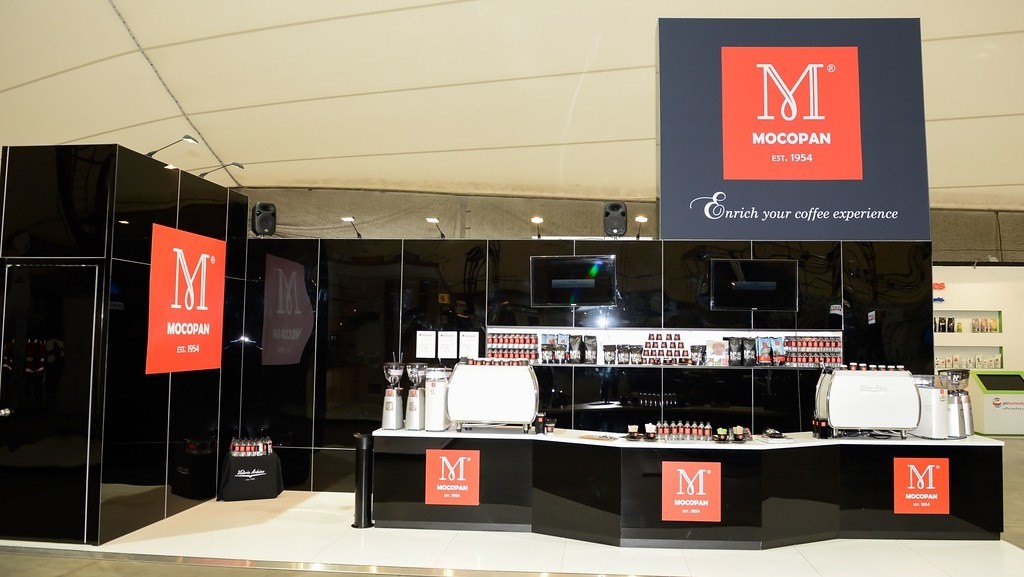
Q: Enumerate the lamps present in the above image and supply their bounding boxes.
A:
[426,217,446,239]
[531,216,544,239]
[973,255,998,269]
[341,216,362,238]
[200,163,245,179]
[145,134,199,158]
[634,215,648,239]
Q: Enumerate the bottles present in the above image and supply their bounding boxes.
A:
[487,348,537,359]
[783,337,841,348]
[229,436,273,458]
[839,362,905,371]
[655,420,712,441]
[466,356,528,366]
[487,334,537,344]
[785,352,842,363]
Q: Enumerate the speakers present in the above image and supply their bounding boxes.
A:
[251,201,277,237]
[604,201,627,237]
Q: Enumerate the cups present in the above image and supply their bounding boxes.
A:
[383,362,405,385]
[545,423,555,433]
[406,362,428,386]
[535,415,546,434]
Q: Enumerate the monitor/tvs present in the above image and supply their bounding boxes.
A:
[709,259,799,312]
[530,254,617,308]
